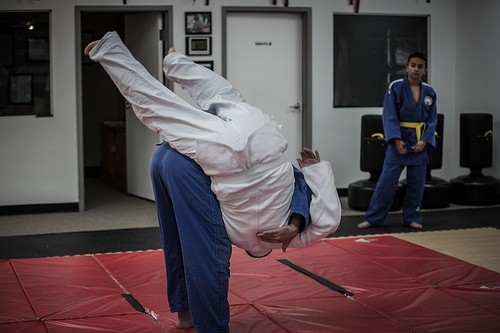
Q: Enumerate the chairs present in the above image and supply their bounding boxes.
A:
[342,60,381,105]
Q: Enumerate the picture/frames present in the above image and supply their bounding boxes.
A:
[194,61,214,72]
[186,37,212,55]
[185,11,212,35]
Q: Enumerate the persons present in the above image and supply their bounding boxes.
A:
[150,140,313,333]
[84,31,342,258]
[358,51,437,228]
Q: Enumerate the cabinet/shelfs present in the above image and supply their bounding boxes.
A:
[101,119,127,192]
